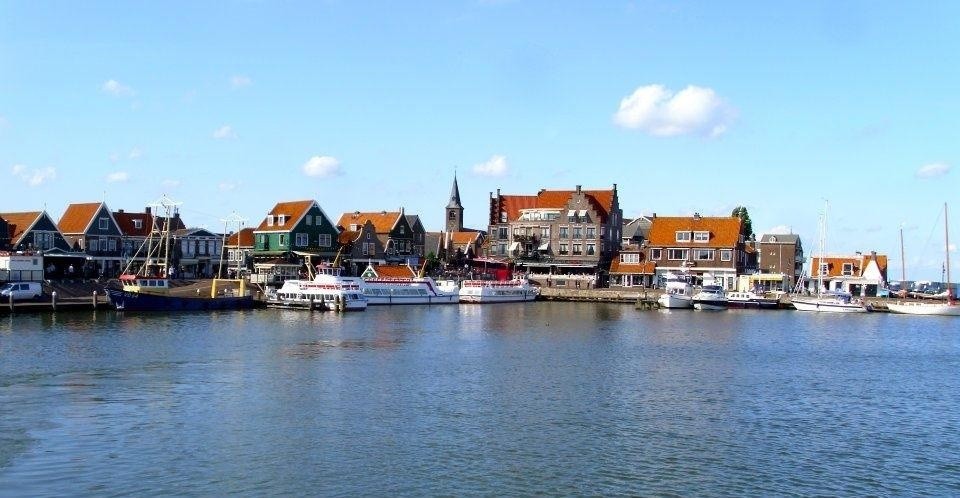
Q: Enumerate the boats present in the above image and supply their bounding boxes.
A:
[658,282,779,310]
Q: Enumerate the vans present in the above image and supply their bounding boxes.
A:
[0,281,42,302]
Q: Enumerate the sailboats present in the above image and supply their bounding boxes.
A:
[791,199,960,315]
